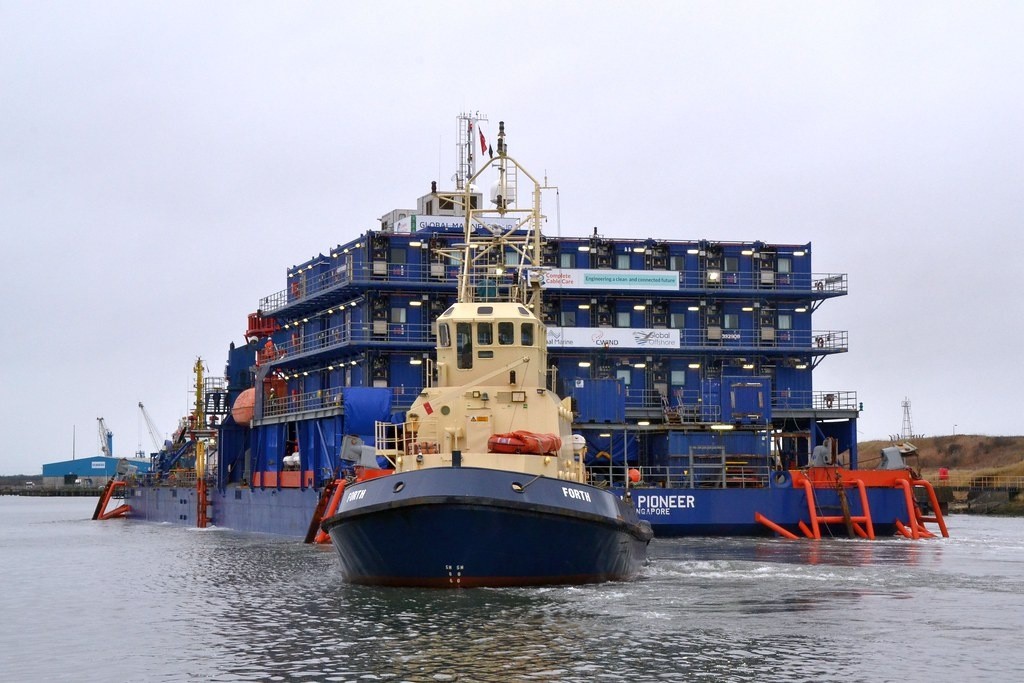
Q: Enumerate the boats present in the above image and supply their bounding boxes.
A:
[318,118,657,586]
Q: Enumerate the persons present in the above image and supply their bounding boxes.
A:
[268,387,277,416]
[213,390,221,413]
[264,337,274,360]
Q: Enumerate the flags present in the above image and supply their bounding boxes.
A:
[479,127,488,156]
